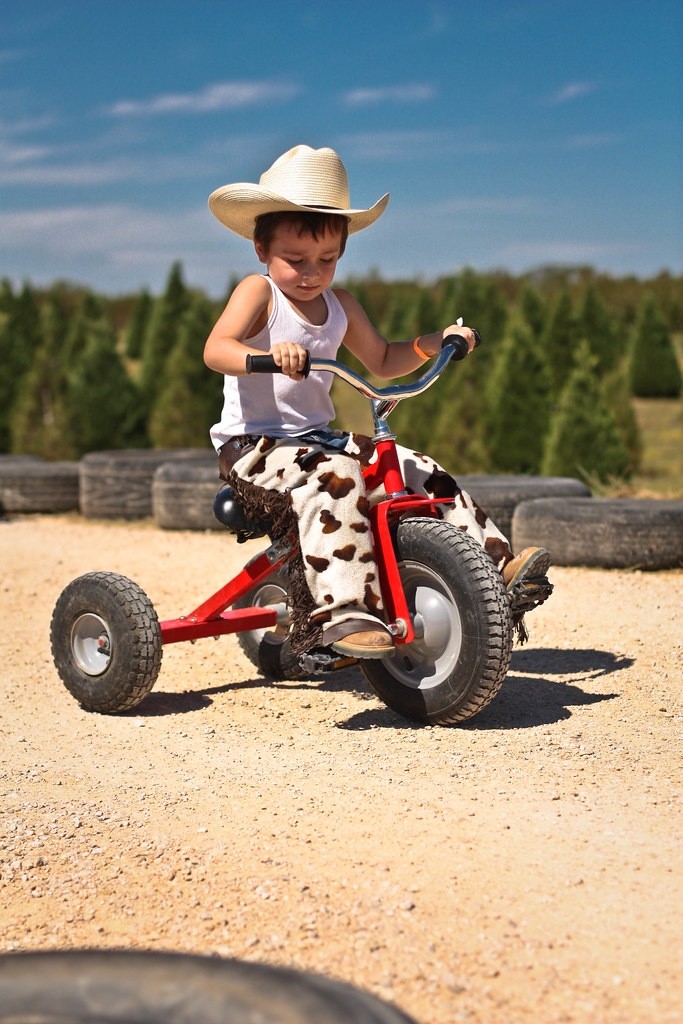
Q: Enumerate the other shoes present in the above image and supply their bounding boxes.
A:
[327,619,395,660]
[500,546,551,627]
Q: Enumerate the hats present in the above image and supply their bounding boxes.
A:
[208,145,390,241]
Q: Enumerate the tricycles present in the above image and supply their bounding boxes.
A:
[46,331,555,731]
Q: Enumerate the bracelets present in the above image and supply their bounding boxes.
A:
[414,336,438,359]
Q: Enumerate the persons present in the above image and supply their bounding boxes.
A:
[203,145,552,660]
